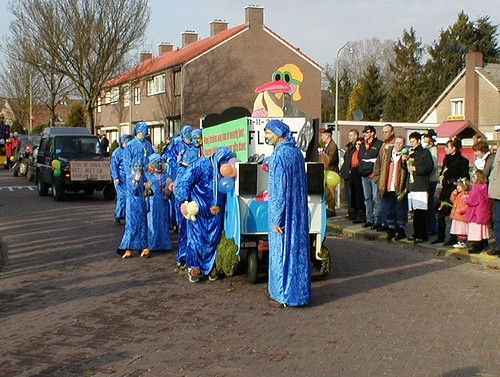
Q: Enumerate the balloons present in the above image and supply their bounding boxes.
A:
[220,164,236,177]
[51,159,60,168]
[218,177,234,193]
[324,170,340,187]
[318,152,330,169]
[10,155,14,161]
[53,168,61,176]
[228,158,241,167]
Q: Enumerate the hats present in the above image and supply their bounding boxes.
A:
[148,154,162,172]
[264,120,290,138]
[324,128,334,134]
[136,122,149,134]
[121,134,129,144]
[181,125,193,139]
[421,129,436,141]
[213,148,234,166]
[192,129,202,147]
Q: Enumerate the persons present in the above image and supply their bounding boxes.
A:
[320,128,340,218]
[5,131,21,169]
[79,143,90,153]
[340,124,500,258]
[96,121,235,284]
[262,120,313,307]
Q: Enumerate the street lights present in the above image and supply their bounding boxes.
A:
[335,40,355,209]
[29,63,46,135]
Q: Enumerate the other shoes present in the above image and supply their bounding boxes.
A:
[430,238,444,244]
[453,242,464,248]
[407,237,416,241]
[487,247,500,255]
[269,299,282,307]
[414,237,424,244]
[468,244,483,254]
[464,243,467,248]
[395,233,406,241]
[483,240,488,251]
[174,263,218,282]
[115,219,150,258]
[326,209,394,239]
[443,238,458,246]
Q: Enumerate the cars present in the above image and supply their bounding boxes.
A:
[0,123,42,182]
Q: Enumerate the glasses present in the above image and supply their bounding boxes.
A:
[363,132,369,134]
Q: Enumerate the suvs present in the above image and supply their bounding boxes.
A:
[35,127,116,201]
